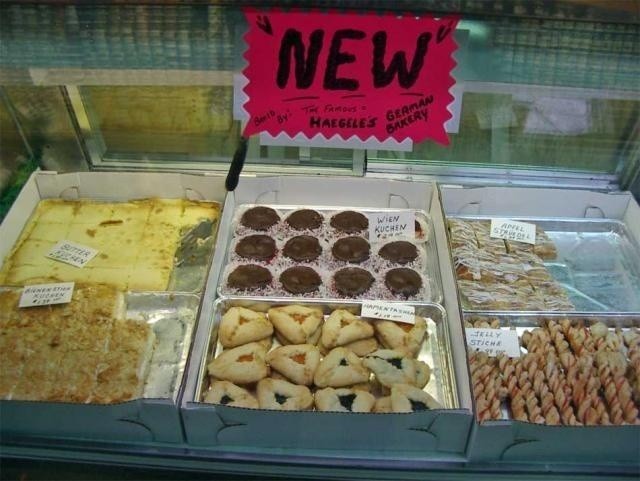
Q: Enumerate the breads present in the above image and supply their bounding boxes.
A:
[447,216,575,311]
[0,280,155,405]
[0,197,224,293]
[224,205,430,303]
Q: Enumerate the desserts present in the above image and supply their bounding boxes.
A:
[201,302,446,412]
